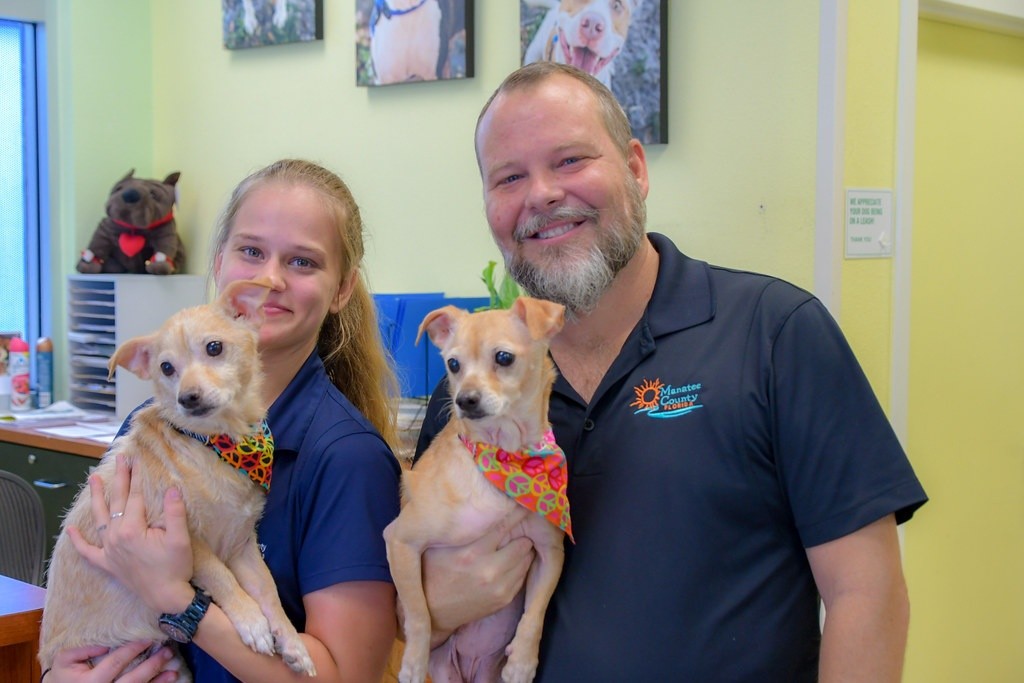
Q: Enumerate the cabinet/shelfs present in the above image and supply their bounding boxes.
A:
[0,414,413,586]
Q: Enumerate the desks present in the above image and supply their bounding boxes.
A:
[0,574,47,683]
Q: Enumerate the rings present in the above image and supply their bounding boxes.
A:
[109,512,124,520]
[96,525,107,533]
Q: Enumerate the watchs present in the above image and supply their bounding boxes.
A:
[157,588,212,646]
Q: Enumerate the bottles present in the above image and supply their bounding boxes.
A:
[34,337,52,409]
[9,336,31,410]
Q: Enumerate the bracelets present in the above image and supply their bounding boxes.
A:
[40,668,51,683]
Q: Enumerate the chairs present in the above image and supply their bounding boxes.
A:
[0,470,47,587]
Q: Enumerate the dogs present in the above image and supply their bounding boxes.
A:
[522,0,634,97]
[384,298,568,683]
[39,279,317,682]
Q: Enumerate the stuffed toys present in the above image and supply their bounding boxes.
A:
[77,170,186,275]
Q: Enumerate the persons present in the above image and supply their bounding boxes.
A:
[394,61,928,683]
[40,158,403,683]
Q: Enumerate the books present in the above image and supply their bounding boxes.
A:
[0,400,126,444]
